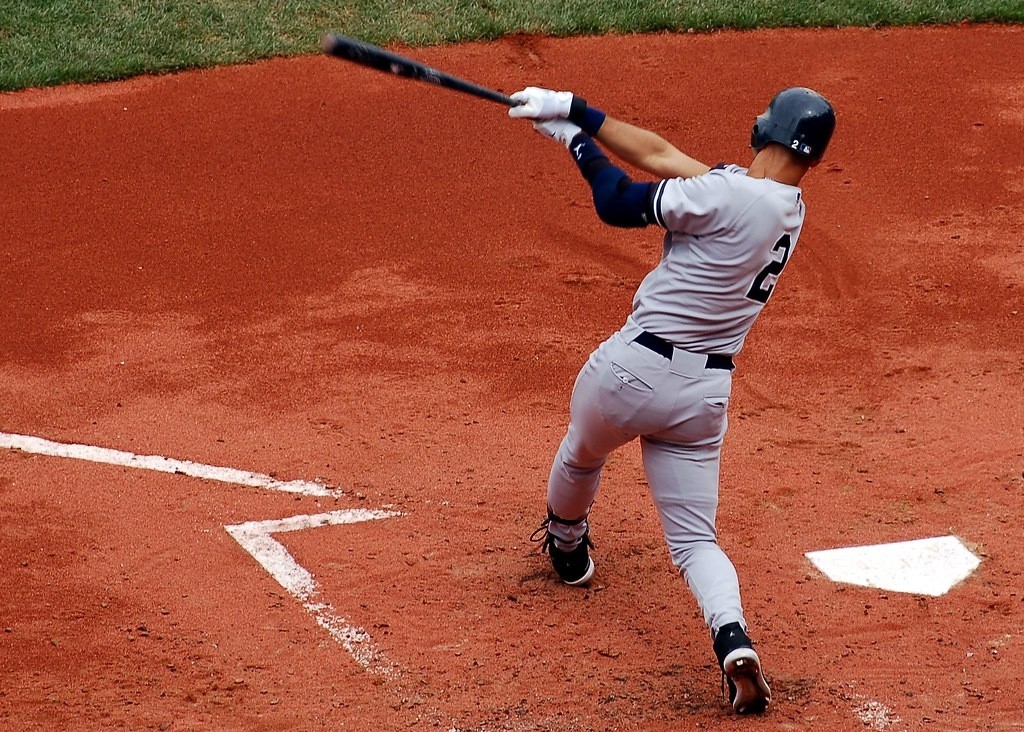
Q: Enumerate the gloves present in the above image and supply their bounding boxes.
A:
[532,119,582,149]
[508,86,573,120]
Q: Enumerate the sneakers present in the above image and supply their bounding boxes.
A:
[542,533,594,586]
[712,622,771,715]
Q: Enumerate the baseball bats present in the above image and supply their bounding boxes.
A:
[320,32,523,106]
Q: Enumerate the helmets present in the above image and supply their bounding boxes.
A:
[751,87,835,157]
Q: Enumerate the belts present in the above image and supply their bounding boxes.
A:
[633,331,735,369]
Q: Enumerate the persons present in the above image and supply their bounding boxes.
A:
[507,87,836,715]
